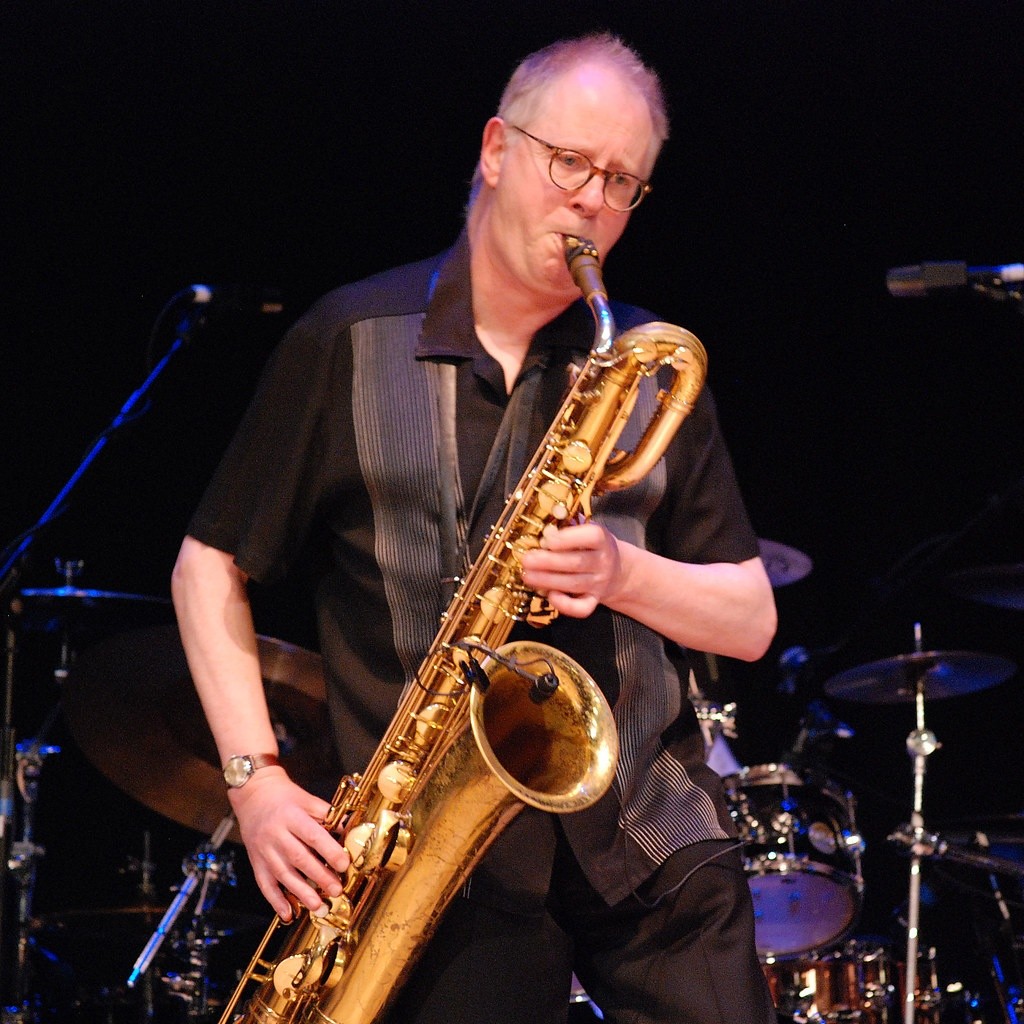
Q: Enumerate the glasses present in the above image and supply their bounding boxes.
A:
[512,125,654,212]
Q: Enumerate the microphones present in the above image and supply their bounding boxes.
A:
[780,646,853,738]
[188,285,284,313]
[888,263,1024,297]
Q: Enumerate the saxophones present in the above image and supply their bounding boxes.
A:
[222,228,710,1023]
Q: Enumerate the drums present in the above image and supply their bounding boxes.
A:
[733,757,860,960]
[763,927,945,1024]
[908,831,1024,1024]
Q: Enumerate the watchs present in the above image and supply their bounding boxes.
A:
[223,753,279,789]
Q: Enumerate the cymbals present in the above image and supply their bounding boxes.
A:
[944,563,1024,619]
[17,581,176,612]
[829,647,1014,706]
[33,891,275,930]
[51,616,375,842]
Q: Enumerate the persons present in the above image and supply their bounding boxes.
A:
[171,34,780,1024]
